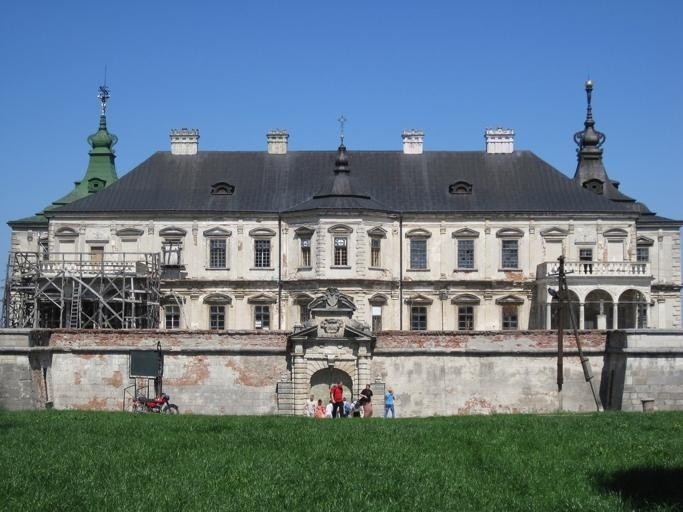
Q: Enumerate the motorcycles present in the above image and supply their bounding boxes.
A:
[130,393,180,415]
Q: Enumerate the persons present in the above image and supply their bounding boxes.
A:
[304,381,373,418]
[385,388,395,418]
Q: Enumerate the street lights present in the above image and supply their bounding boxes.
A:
[547,254,568,410]
